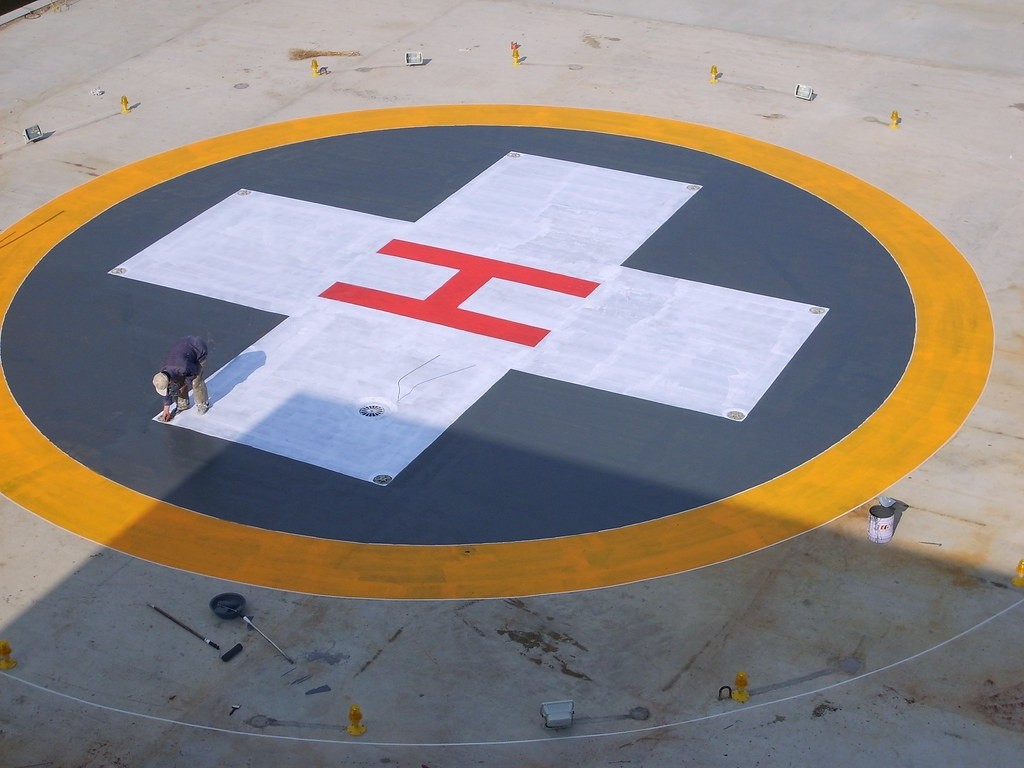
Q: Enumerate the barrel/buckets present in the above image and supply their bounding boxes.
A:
[866,505,894,543]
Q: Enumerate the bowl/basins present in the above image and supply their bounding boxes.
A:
[209,593,246,619]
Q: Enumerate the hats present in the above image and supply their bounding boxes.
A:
[153,372,169,397]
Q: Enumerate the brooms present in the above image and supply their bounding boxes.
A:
[289,47,360,61]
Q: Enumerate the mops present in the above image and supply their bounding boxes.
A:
[145,602,243,662]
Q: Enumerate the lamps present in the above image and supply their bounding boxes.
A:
[405,52,423,66]
[23,125,43,144]
[540,700,575,732]
[795,84,815,102]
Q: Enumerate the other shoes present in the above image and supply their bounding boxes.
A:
[173,405,191,413]
[197,402,209,415]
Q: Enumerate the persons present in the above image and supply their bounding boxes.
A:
[152,334,209,423]
